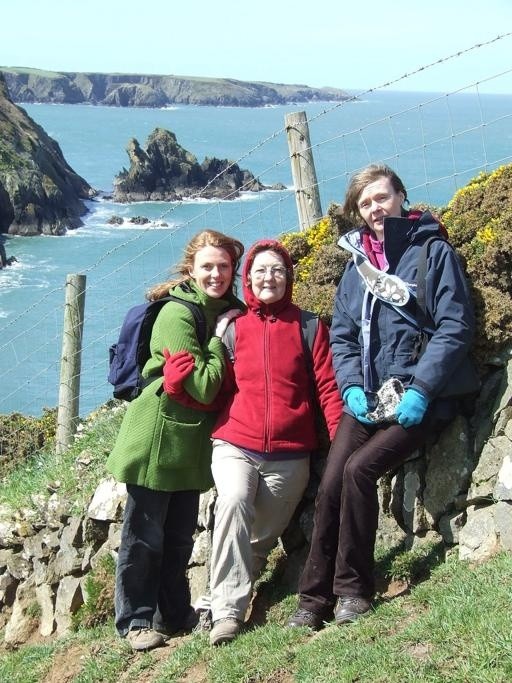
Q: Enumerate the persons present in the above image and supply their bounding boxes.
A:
[284,165,482,631]
[107,230,246,650]
[163,240,342,648]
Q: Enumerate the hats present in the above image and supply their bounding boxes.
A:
[366,378,406,424]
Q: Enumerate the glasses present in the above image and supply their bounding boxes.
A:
[252,265,287,279]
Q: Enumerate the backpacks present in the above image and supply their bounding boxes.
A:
[107,296,207,401]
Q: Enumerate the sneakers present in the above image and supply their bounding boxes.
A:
[209,617,243,644]
[285,605,325,630]
[127,626,164,652]
[335,595,374,624]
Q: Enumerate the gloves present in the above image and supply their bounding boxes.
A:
[342,386,374,425]
[394,390,429,428]
[162,346,195,393]
